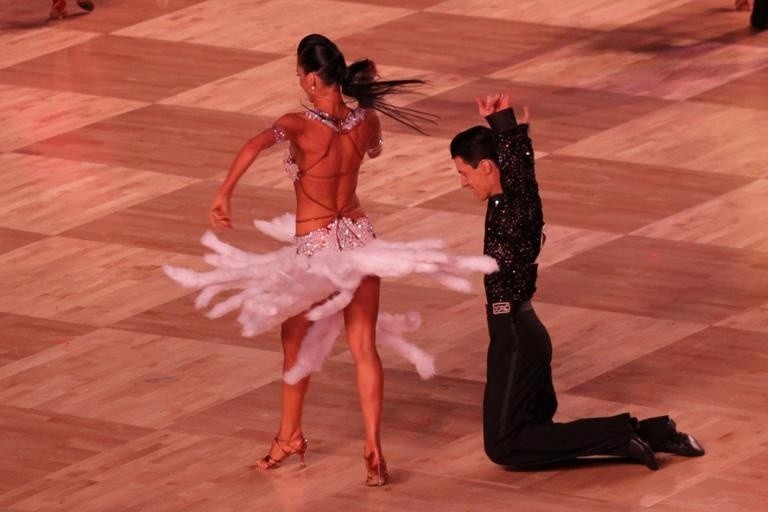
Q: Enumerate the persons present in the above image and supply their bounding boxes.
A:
[448,94,704,471]
[209,34,440,486]
[48,1,94,17]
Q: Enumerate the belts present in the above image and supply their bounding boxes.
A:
[520,300,533,312]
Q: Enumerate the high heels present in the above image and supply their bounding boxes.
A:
[366,448,390,486]
[257,432,308,469]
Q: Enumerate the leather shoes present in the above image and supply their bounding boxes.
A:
[627,416,658,470]
[660,415,703,456]
